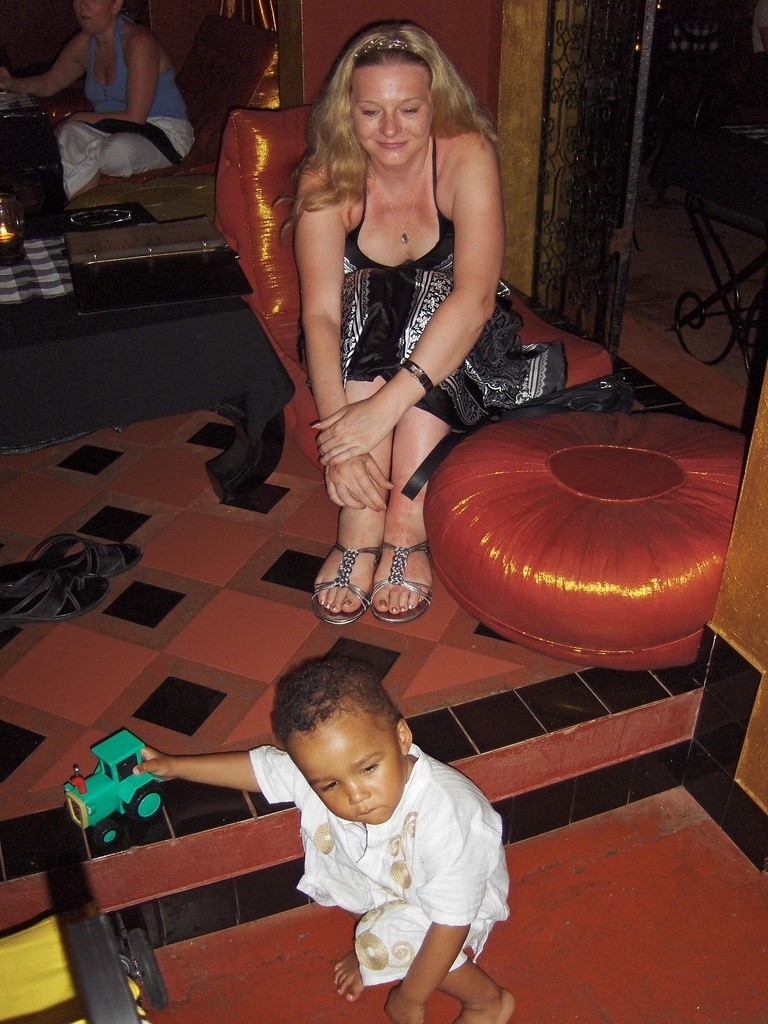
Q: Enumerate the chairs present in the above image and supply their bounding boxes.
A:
[214,101,613,475]
[97,14,279,186]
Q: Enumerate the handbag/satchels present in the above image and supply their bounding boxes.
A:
[477,372,636,428]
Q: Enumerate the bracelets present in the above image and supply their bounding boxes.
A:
[401,359,435,393]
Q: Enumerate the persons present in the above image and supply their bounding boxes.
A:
[273,21,567,624]
[133,655,516,1024]
[0,0,195,200]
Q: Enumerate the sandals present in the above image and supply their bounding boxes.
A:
[311,544,382,624]
[370,540,434,622]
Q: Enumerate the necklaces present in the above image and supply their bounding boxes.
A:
[366,157,429,244]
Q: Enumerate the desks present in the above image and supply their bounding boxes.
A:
[671,186,768,381]
[0,261,285,502]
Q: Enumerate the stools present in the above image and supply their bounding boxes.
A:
[423,413,748,672]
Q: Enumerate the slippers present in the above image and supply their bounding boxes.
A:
[0,571,113,631]
[0,533,143,578]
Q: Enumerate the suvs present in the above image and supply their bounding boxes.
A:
[61,728,169,849]
